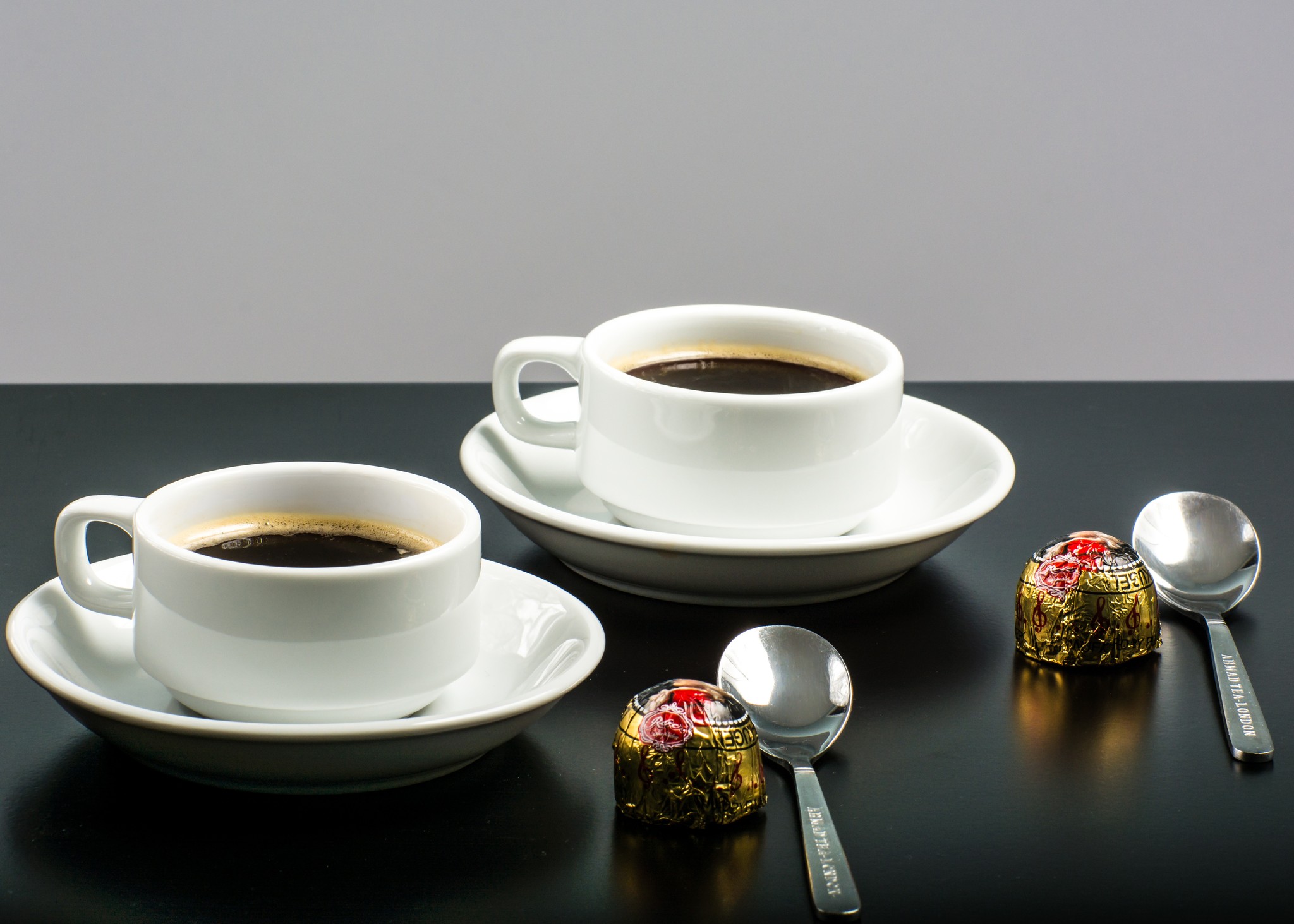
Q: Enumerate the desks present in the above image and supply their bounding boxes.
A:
[0,384,1294,924]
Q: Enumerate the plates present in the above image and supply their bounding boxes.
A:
[4,552,606,795]
[459,386,1017,606]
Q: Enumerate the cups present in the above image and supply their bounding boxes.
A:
[54,460,482,721]
[492,304,904,536]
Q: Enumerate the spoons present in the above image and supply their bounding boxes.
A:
[1132,491,1274,763]
[717,624,861,915]
